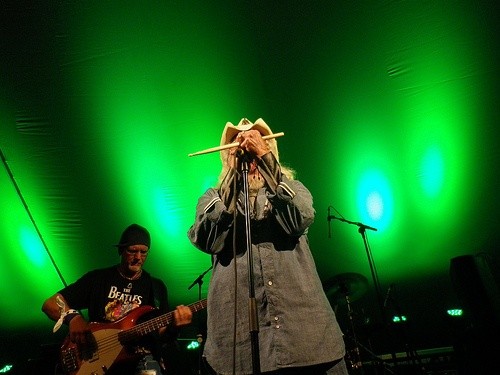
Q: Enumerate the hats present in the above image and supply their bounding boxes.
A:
[220,117,278,166]
[118,224,150,253]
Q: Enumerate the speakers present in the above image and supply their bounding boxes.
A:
[177,338,202,375]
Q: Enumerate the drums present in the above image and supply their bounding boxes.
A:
[344,347,362,369]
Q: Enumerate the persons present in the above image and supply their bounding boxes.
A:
[186,117,351,375]
[40,223,192,375]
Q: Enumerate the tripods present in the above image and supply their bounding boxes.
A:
[340,293,396,375]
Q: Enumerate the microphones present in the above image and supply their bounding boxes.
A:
[236,148,245,156]
[384,283,393,306]
[328,207,331,237]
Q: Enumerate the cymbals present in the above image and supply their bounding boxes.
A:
[323,272,371,307]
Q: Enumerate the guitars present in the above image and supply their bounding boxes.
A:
[59,296,208,375]
